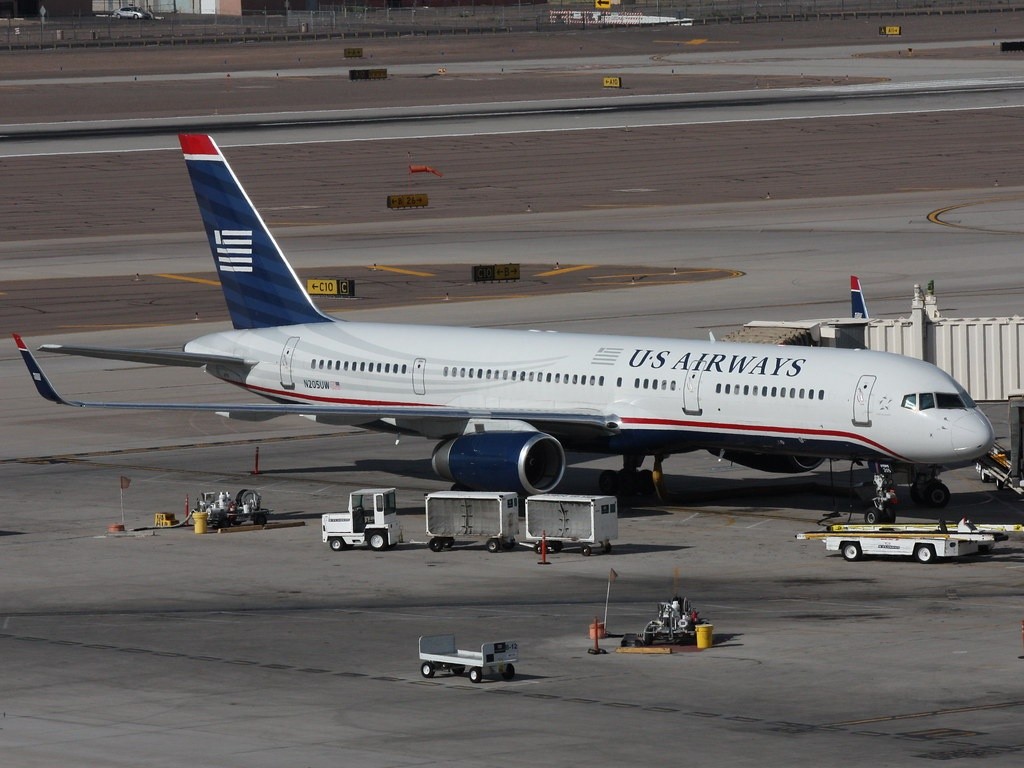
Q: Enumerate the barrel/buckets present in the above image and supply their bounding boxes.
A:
[694,624,714,649]
[192,512,209,534]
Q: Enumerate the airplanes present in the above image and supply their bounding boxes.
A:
[12,133,996,525]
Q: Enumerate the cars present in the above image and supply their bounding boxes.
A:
[113,5,151,20]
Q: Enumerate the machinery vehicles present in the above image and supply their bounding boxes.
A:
[321,487,403,551]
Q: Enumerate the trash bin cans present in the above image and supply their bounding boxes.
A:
[192,511,208,534]
[694,623,713,648]
